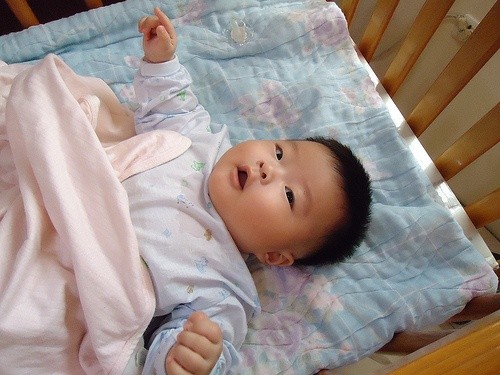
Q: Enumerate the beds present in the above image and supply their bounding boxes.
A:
[0,0,500,375]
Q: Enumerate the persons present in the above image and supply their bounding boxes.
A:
[95,2,375,375]
